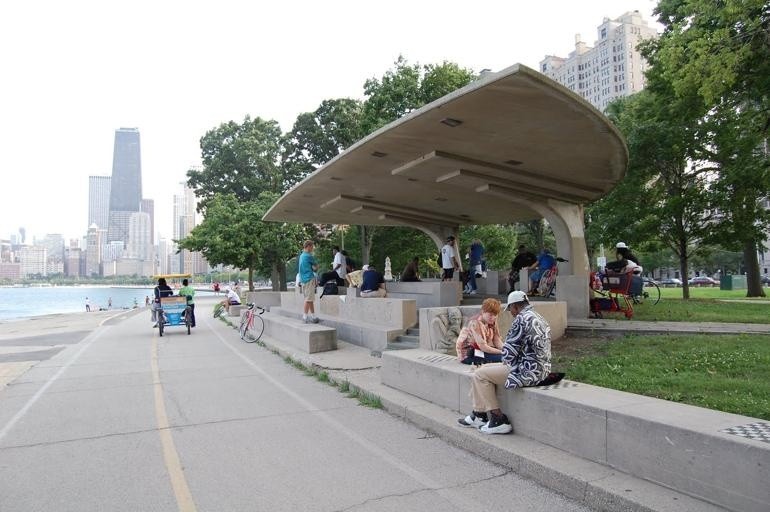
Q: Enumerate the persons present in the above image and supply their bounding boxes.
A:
[441,236,458,281]
[346,264,369,287]
[463,252,488,294]
[358,265,387,297]
[212,281,241,316]
[471,238,484,268]
[457,289,551,435]
[616,241,642,301]
[134,294,154,307]
[455,298,503,365]
[398,256,422,282]
[151,278,173,322]
[180,279,195,326]
[506,245,537,295]
[295,239,352,324]
[108,297,112,309]
[525,249,554,296]
[85,296,90,312]
[432,307,468,355]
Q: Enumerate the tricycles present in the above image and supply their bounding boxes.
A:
[152,273,193,336]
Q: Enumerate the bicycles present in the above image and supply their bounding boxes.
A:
[213,302,225,319]
[630,280,660,307]
[536,257,569,299]
[239,302,265,344]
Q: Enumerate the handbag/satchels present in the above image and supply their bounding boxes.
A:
[438,252,442,268]
[465,344,485,358]
[323,283,338,295]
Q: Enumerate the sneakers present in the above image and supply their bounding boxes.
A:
[457,412,488,432]
[302,316,319,323]
[222,312,229,317]
[153,322,158,328]
[479,413,512,434]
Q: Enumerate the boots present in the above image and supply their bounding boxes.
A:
[462,282,478,294]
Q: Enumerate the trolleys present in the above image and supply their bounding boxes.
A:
[589,265,639,320]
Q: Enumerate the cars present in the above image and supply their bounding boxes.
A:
[659,278,683,287]
[687,276,721,287]
[642,277,660,287]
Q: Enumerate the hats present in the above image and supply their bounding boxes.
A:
[363,264,376,271]
[544,249,550,253]
[447,236,455,242]
[503,291,531,312]
[519,245,526,249]
[616,242,628,248]
